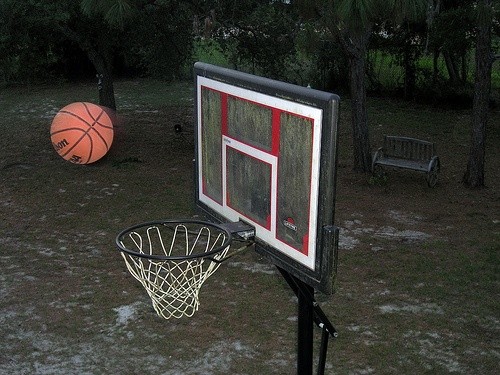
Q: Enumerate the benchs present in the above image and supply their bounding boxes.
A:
[370,134,440,189]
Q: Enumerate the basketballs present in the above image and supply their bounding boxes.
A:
[50,101,114,164]
